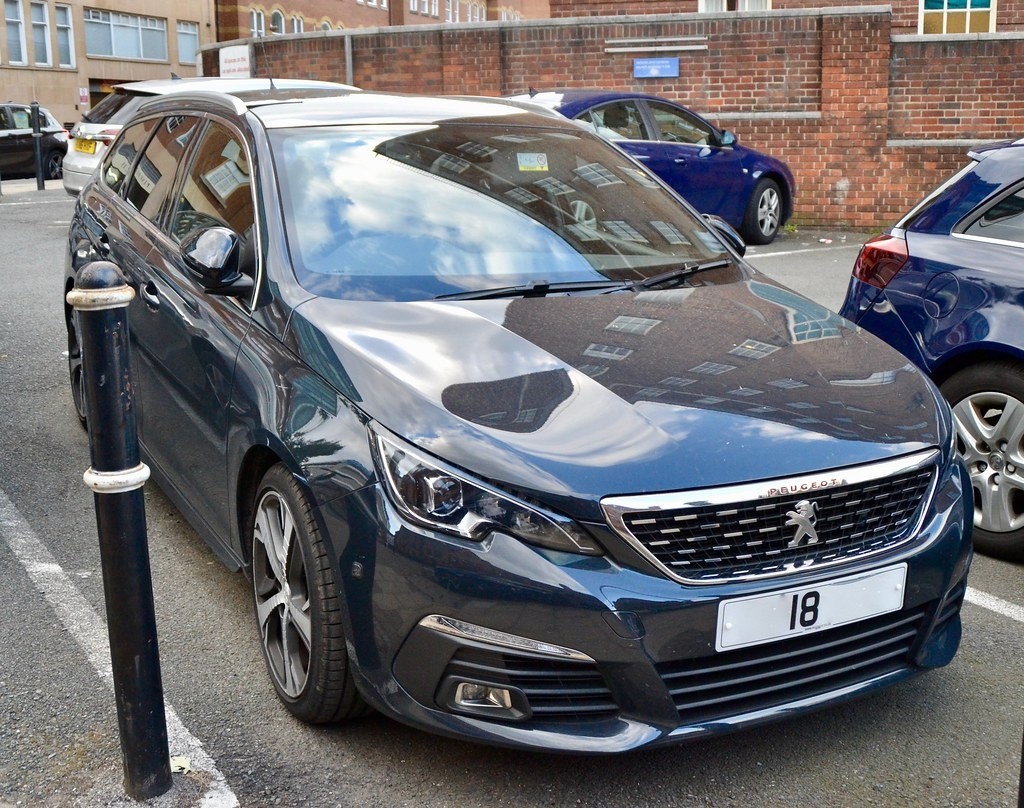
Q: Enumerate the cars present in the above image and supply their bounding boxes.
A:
[62,84,974,756]
[837,137,1024,567]
[0,104,71,181]
[492,86,796,244]
[60,78,363,197]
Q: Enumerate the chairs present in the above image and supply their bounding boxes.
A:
[602,106,630,130]
